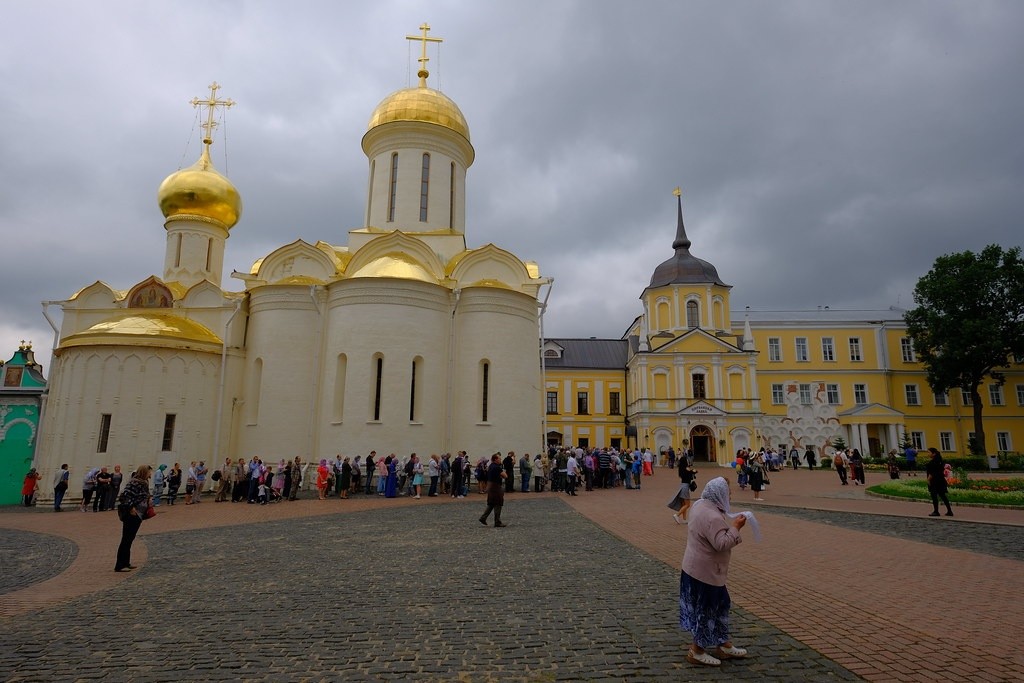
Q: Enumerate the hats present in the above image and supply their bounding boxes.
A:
[749,451,756,459]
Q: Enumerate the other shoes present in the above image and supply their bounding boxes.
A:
[151,483,644,508]
[128,566,137,569]
[673,514,680,525]
[495,523,506,527]
[855,480,858,485]
[478,518,487,526]
[929,511,940,516]
[754,498,764,502]
[842,481,848,485]
[681,519,688,523]
[116,568,130,572]
[945,511,953,516]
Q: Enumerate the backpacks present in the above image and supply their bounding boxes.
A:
[851,457,861,467]
[834,451,843,466]
[474,468,481,480]
[451,459,461,473]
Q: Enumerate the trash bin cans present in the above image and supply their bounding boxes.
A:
[653,454,657,463]
[988,455,999,471]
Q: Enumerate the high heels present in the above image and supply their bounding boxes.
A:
[686,648,721,666]
[718,644,747,660]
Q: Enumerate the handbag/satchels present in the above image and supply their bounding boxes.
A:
[118,504,129,522]
[689,481,697,492]
[143,507,155,520]
[763,474,769,486]
[33,484,39,491]
[212,471,221,481]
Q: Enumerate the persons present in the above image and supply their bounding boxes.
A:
[113,464,154,574]
[887,451,900,481]
[540,442,656,496]
[789,446,799,470]
[666,445,699,525]
[79,465,123,513]
[185,460,209,504]
[152,464,166,507]
[54,464,70,511]
[21,468,43,508]
[502,451,516,493]
[679,475,749,665]
[479,454,508,529]
[736,444,784,502]
[834,445,868,487]
[212,455,303,506]
[167,463,183,506]
[518,454,533,493]
[804,448,815,470]
[535,454,546,493]
[905,444,918,476]
[315,450,491,499]
[925,448,954,516]
